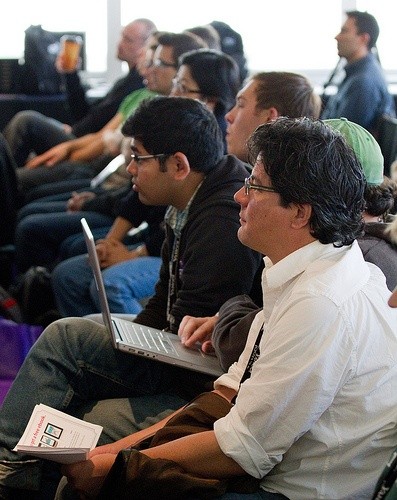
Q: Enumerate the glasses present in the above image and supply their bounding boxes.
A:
[155,58,178,70]
[130,151,166,162]
[243,177,281,196]
[171,78,206,94]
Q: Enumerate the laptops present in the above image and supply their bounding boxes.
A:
[79,218,225,376]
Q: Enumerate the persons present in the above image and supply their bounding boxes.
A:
[1,10,396,500]
[59,117,397,500]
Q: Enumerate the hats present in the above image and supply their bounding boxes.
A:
[314,116,384,185]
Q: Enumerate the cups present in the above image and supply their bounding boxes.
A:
[60,35,83,73]
